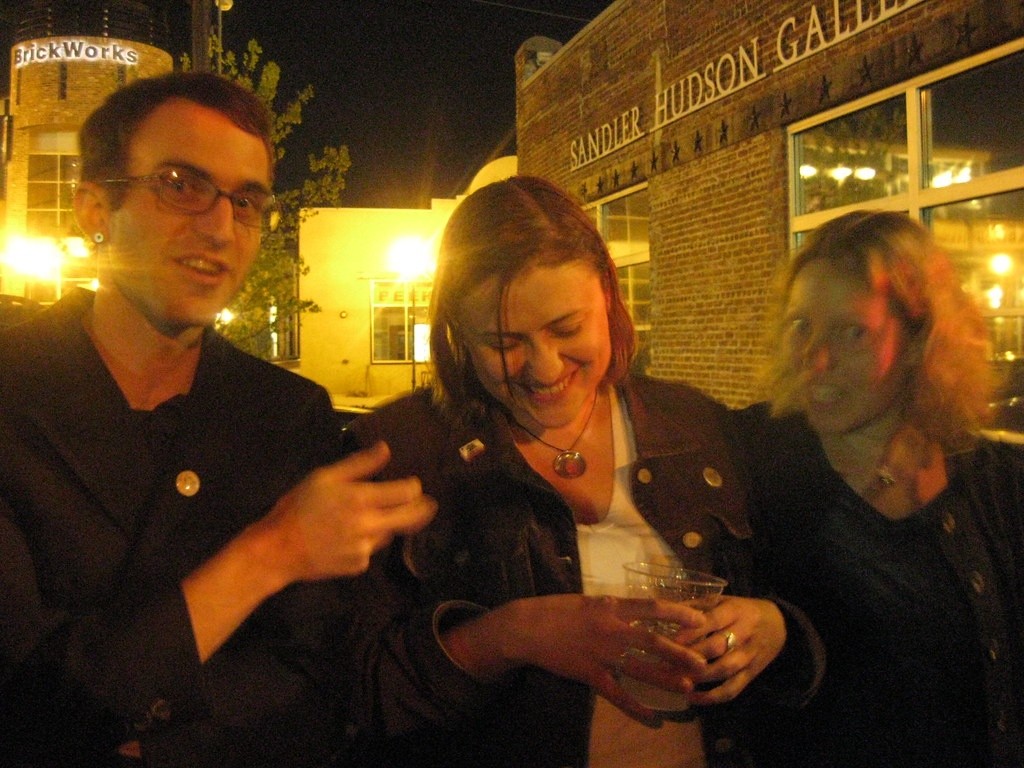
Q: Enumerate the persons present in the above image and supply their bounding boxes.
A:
[1,72,436,768]
[320,172,847,768]
[732,208,1024,768]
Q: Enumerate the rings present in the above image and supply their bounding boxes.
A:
[877,468,897,487]
[721,630,737,651]
[614,652,632,676]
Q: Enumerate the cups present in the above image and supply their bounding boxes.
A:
[614,561,729,712]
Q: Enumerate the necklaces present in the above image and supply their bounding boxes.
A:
[516,387,603,479]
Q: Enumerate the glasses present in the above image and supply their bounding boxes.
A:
[102,171,282,228]
